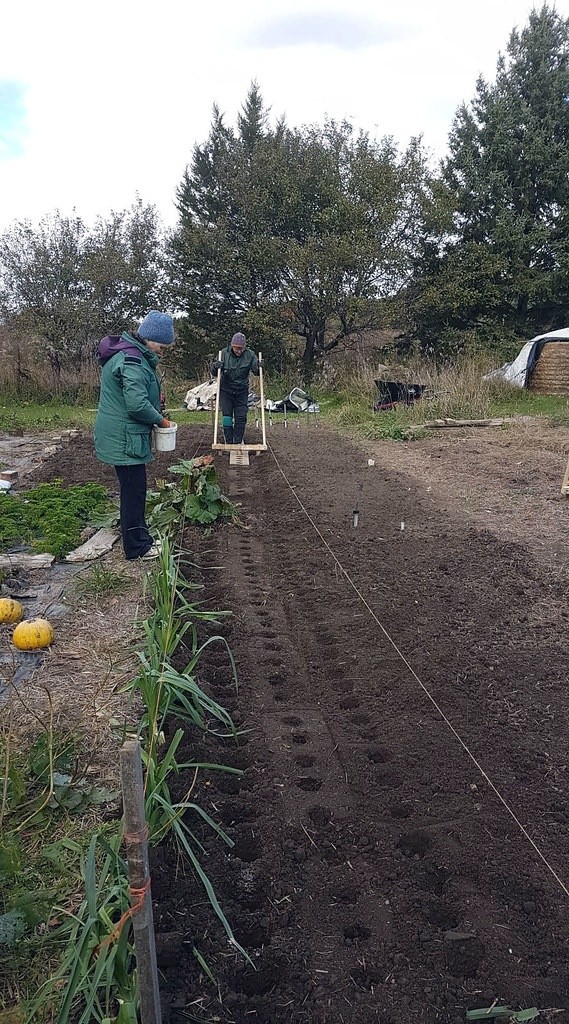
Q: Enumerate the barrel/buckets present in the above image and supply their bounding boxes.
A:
[153,420,177,452]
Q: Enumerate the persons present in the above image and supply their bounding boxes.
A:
[93,310,178,560]
[209,332,266,445]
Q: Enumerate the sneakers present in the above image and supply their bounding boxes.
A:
[133,546,162,561]
[152,540,163,546]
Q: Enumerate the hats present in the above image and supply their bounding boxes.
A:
[231,332,245,347]
[138,310,174,345]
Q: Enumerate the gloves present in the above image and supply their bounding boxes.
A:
[257,358,265,369]
[214,361,224,369]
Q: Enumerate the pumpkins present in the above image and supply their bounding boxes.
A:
[13,616,54,650]
[0,596,24,623]
